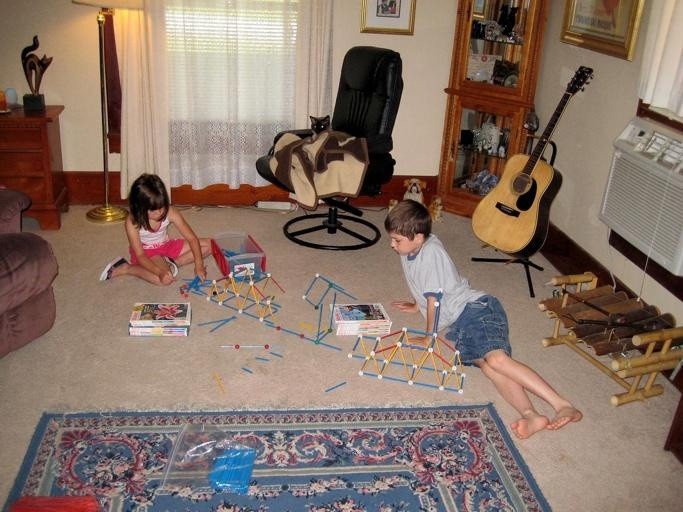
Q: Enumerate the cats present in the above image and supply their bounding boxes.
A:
[309,115,331,141]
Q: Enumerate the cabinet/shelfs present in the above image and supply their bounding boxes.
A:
[437,0,550,219]
[0,102,65,231]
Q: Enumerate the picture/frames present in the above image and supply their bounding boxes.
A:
[558,1,645,62]
[359,0,416,36]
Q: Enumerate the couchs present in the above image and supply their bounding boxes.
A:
[0,189,58,359]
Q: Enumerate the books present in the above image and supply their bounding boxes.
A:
[330,302,392,336]
[128,302,191,337]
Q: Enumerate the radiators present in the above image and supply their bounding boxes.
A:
[599,116,683,277]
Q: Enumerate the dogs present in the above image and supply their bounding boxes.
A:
[402,178,427,205]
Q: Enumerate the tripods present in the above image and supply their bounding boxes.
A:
[470,112,544,297]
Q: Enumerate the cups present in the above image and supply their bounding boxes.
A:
[5,88,17,107]
[0,92,7,111]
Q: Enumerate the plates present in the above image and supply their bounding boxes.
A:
[0,109,12,114]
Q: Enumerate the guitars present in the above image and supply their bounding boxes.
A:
[472,66,594,259]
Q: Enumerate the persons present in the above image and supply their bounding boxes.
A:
[384,199,583,439]
[99,173,212,285]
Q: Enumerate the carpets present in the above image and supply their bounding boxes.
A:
[5,403,552,512]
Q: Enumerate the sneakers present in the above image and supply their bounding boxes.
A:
[162,256,179,277]
[98,256,127,281]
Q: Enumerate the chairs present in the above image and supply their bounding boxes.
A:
[256,46,404,251]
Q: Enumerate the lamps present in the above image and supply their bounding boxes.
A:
[72,1,146,222]
